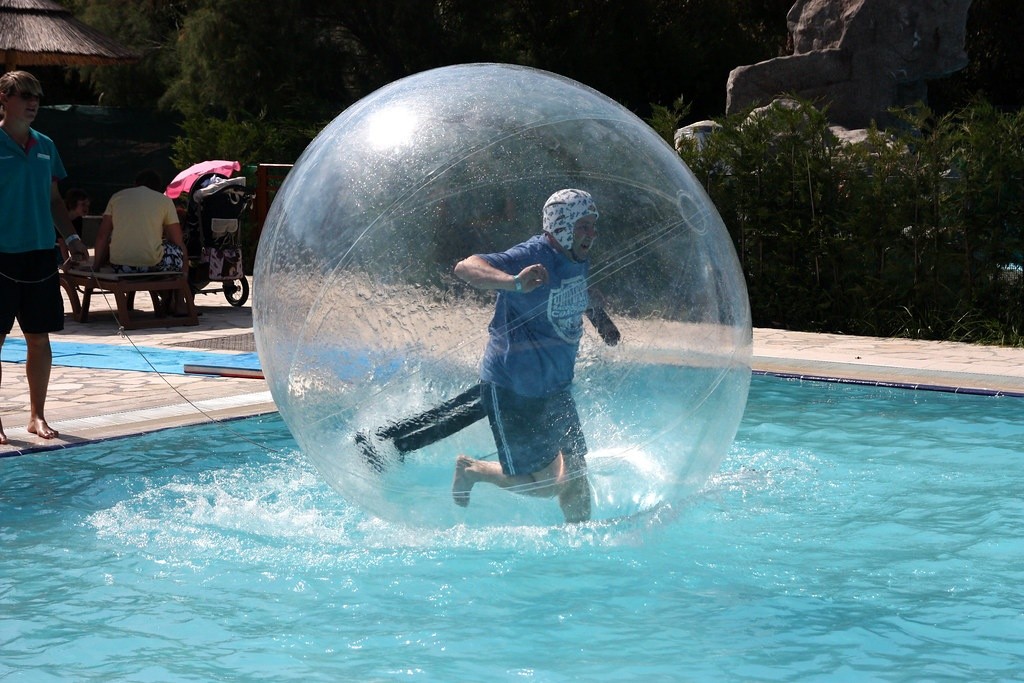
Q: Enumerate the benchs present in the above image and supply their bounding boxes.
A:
[58,261,199,331]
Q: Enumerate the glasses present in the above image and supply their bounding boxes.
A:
[18,91,40,102]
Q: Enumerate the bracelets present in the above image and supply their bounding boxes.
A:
[65,234,81,246]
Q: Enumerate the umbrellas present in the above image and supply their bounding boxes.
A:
[164,159,241,201]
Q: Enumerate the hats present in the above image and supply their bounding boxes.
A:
[542,189,599,251]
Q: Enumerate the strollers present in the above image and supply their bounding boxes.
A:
[156,158,259,307]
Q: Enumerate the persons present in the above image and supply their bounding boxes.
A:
[90,167,204,318]
[451,188,620,525]
[0,71,93,442]
[56,190,87,265]
